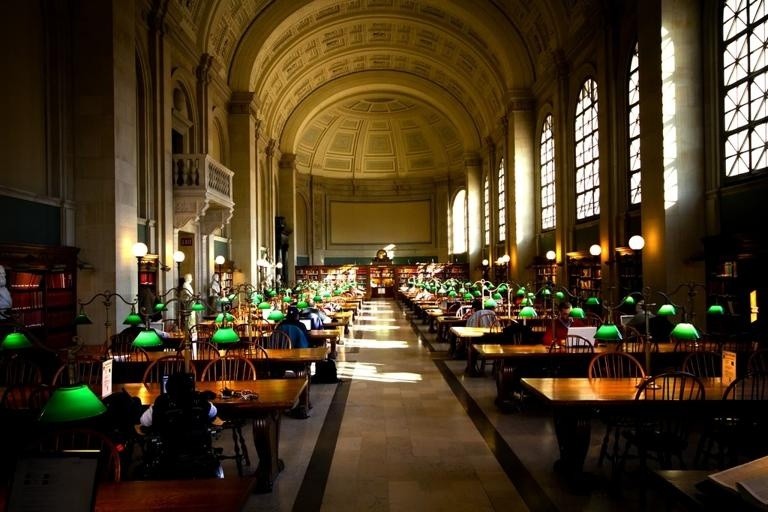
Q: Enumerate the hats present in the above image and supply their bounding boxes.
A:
[286,307,298,318]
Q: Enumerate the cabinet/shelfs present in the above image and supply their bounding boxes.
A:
[394,264,467,300]
[295,264,372,301]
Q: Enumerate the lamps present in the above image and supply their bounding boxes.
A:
[257,259,269,272]
[174,251,185,290]
[546,251,555,289]
[498,255,510,270]
[216,255,225,295]
[589,245,601,297]
[629,235,645,312]
[134,242,147,316]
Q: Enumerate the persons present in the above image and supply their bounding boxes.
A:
[211,274,220,297]
[543,302,585,378]
[183,274,195,305]
[271,307,310,371]
[0,265,12,318]
[345,287,356,296]
[307,308,325,328]
[462,300,481,319]
[139,374,218,447]
[466,303,501,327]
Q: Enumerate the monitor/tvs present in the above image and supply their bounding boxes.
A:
[159,374,198,394]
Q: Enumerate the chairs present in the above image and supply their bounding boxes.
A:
[0,286,363,495]
[400,276,768,482]
